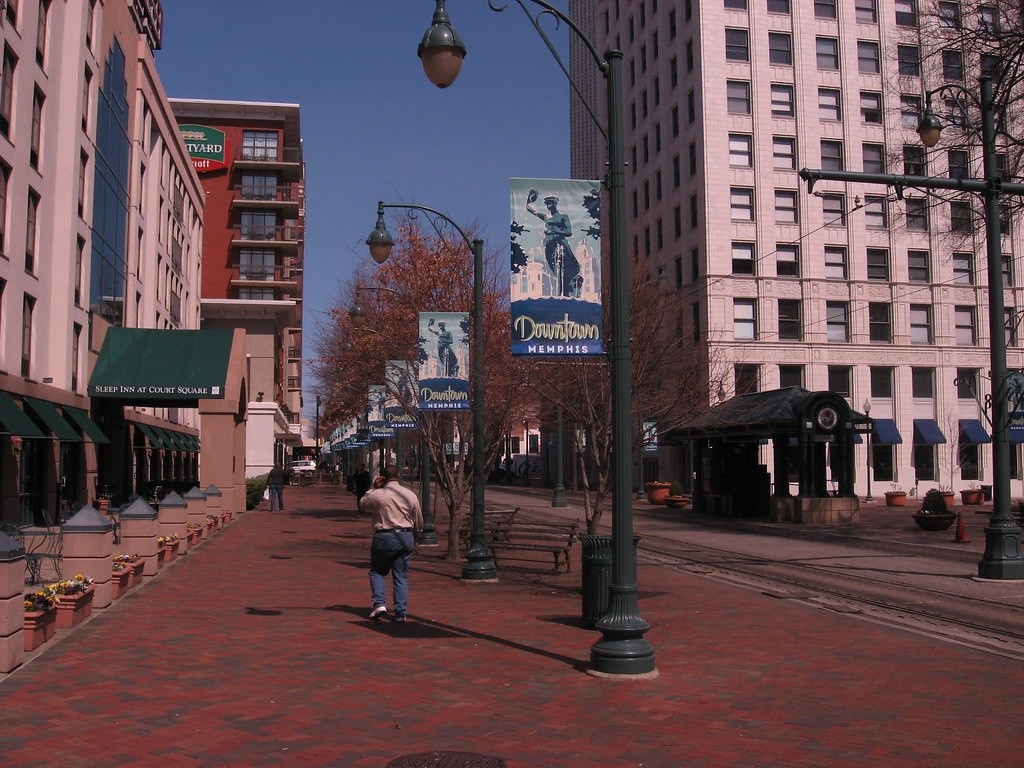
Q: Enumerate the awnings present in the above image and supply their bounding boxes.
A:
[23,396,84,443]
[959,420,991,443]
[131,424,201,453]
[0,390,48,438]
[62,404,112,444]
[913,419,946,444]
[1008,411,1024,443]
[876,420,902,444]
[855,435,863,444]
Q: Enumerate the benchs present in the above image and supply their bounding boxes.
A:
[447,507,581,580]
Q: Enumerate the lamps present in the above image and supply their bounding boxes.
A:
[258,392,264,398]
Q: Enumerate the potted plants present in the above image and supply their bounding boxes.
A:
[187,523,203,544]
[663,481,690,510]
[884,482,985,532]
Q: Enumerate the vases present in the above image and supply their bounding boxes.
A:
[187,531,193,549]
[159,540,180,567]
[220,511,233,527]
[112,559,146,600]
[645,482,671,505]
[23,584,96,652]
[206,519,218,536]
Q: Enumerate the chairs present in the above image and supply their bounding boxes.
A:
[248,230,274,240]
[247,192,272,200]
[0,509,64,587]
[245,269,267,280]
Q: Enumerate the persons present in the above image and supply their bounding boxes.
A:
[353,464,371,512]
[359,466,422,621]
[268,463,287,511]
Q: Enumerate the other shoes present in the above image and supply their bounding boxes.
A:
[395,615,406,622]
[369,606,387,620]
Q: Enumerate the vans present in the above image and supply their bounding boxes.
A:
[285,460,316,473]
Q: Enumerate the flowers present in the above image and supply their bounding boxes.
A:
[24,573,94,613]
[647,481,670,484]
[157,533,180,549]
[113,553,139,571]
[206,514,215,519]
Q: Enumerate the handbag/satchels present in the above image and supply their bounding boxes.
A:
[263,488,269,501]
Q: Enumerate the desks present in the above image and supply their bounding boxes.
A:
[7,530,55,584]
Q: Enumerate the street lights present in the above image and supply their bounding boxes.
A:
[365,200,503,585]
[861,397,878,504]
[348,279,440,547]
[416,0,661,682]
[915,52,1023,582]
[316,395,323,471]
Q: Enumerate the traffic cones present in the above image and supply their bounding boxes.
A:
[949,512,972,543]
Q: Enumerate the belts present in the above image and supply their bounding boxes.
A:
[376,528,412,534]
